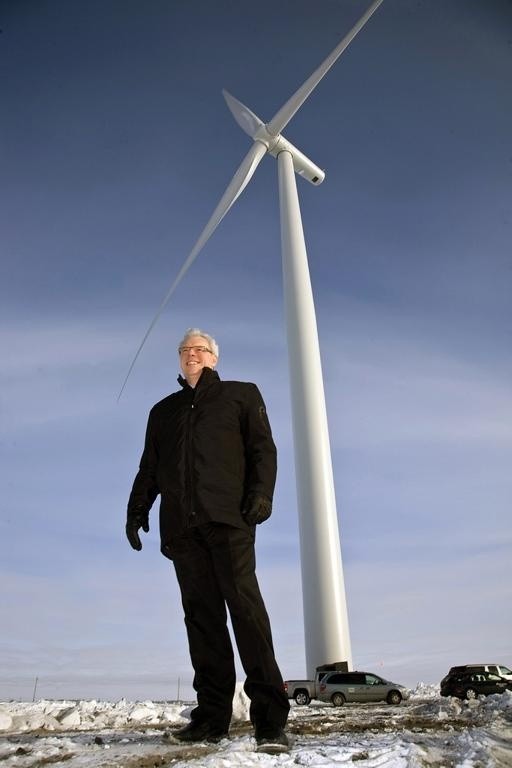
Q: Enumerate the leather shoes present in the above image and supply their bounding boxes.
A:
[162,718,231,746]
[254,726,289,752]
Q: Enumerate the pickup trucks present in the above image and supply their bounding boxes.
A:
[285,670,356,705]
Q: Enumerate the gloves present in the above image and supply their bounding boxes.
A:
[241,490,271,526]
[126,500,151,551]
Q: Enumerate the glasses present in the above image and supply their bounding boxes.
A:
[178,346,212,355]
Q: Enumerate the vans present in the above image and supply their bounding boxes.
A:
[442,663,512,687]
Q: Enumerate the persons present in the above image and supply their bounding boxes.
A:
[123,325,292,753]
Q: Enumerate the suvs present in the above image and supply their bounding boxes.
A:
[442,674,509,700]
[320,672,410,704]
